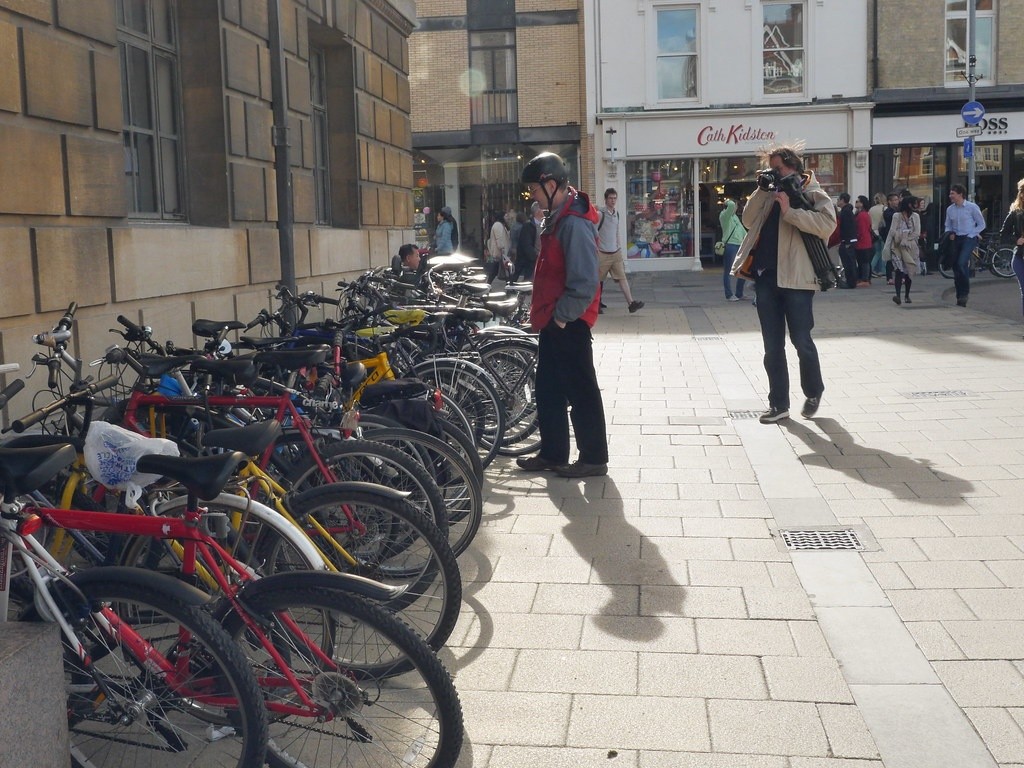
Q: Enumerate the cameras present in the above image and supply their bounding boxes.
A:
[757,168,779,192]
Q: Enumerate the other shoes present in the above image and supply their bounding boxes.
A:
[726,295,739,301]
[905,297,912,303]
[956,295,968,307]
[841,283,856,289]
[893,297,901,304]
[601,303,607,308]
[628,301,644,313]
[888,279,895,285]
[871,272,879,278]
[857,281,871,287]
[739,295,750,300]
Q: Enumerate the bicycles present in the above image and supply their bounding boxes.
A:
[936,233,1016,280]
[0,249,540,768]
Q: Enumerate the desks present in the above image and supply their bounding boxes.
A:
[690,229,716,263]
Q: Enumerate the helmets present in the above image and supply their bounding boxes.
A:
[522,151,568,184]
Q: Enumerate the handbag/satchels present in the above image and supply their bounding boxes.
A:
[871,229,879,243]
[879,227,887,243]
[498,254,515,279]
[714,242,724,256]
[919,246,934,262]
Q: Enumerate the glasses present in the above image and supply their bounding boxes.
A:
[856,201,861,203]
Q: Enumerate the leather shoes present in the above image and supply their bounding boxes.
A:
[516,457,568,471]
[551,460,608,478]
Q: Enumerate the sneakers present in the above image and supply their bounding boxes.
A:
[800,392,822,418]
[759,407,789,422]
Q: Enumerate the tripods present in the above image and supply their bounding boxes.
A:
[779,172,842,291]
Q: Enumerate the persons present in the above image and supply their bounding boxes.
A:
[515,151,610,480]
[485,189,545,294]
[727,137,837,425]
[945,182,987,307]
[881,196,922,305]
[999,178,1024,315]
[836,188,938,289]
[596,184,646,313]
[398,241,422,284]
[719,195,757,304]
[432,204,461,257]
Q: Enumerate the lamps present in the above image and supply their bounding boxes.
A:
[483,148,487,155]
[516,148,522,159]
[494,147,499,155]
[508,148,514,154]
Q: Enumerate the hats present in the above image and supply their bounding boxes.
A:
[442,207,451,215]
